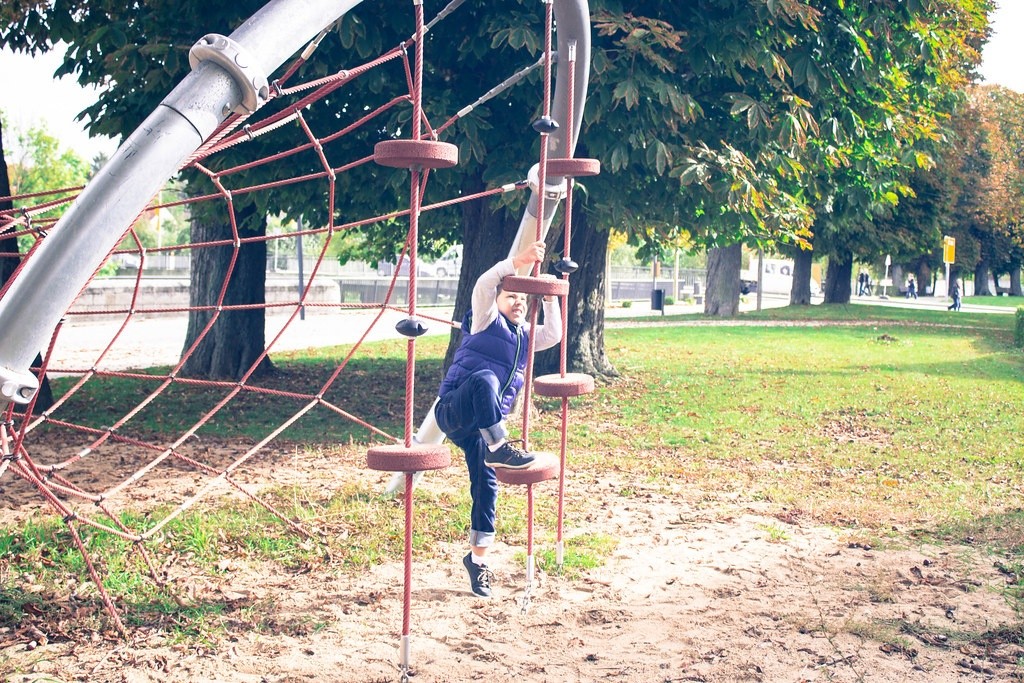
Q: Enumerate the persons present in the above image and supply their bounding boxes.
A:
[858,269,872,296]
[906,279,917,298]
[948,279,962,311]
[434,241,564,597]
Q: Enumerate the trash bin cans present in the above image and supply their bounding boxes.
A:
[651,289,665,310]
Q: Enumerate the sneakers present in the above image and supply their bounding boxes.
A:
[463,550,495,599]
[484,440,536,470]
